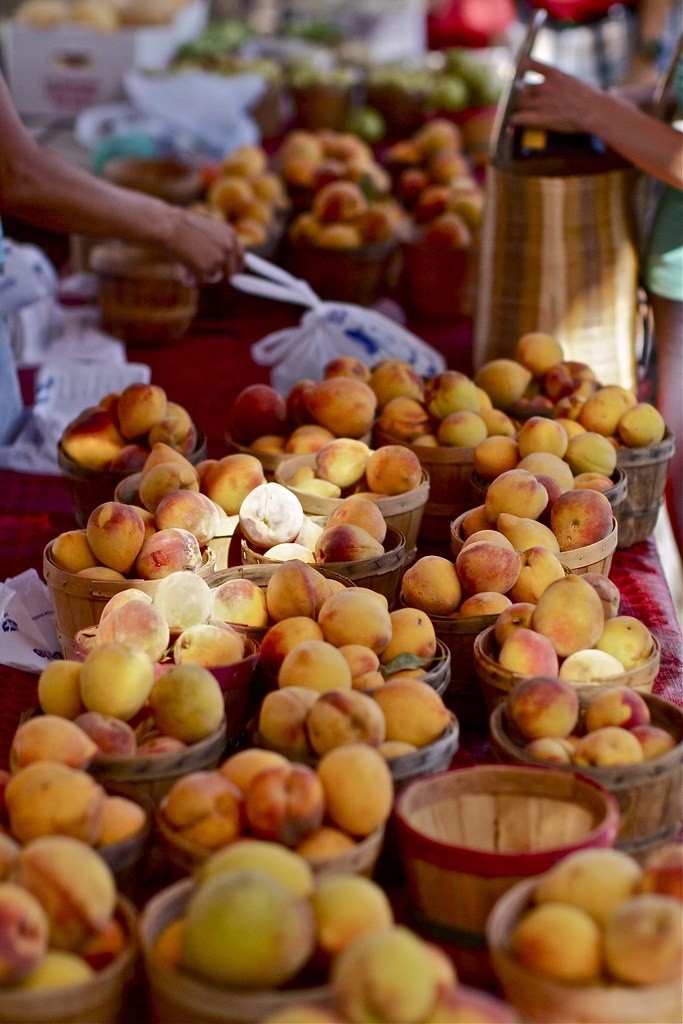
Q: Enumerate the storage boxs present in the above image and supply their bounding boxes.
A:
[0,17,133,120]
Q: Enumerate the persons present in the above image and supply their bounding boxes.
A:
[0,0,245,283]
[509,0,683,562]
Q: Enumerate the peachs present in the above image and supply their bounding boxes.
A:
[0,333,683,1024]
[180,117,490,250]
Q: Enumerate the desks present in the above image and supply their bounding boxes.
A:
[0,167,683,1023]
